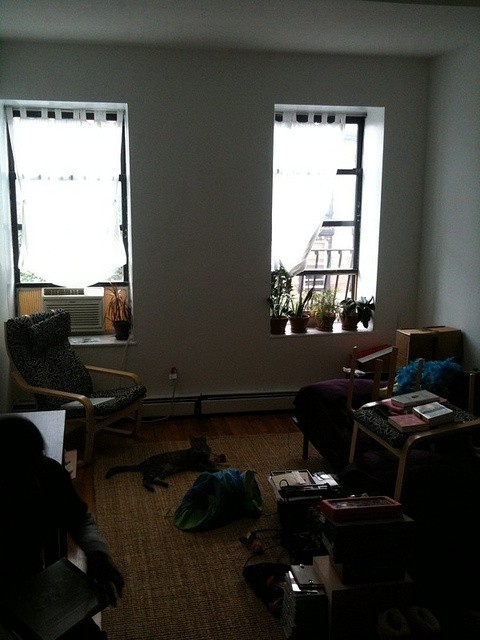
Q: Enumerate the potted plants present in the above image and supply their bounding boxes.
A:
[105,284,132,340]
[285,288,314,333]
[267,262,295,335]
[314,289,340,332]
[339,297,374,331]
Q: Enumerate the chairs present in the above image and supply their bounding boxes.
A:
[4,308,144,467]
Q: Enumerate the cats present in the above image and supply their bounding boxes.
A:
[104,432,231,492]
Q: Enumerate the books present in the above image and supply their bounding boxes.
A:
[386,388,454,434]
[281,565,328,639]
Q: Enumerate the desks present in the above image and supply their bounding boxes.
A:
[0,410,66,472]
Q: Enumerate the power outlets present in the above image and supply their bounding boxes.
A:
[170,367,182,385]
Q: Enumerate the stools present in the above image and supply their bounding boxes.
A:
[349,389,480,500]
[292,377,395,468]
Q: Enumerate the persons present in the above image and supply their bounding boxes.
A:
[0,413,125,640]
